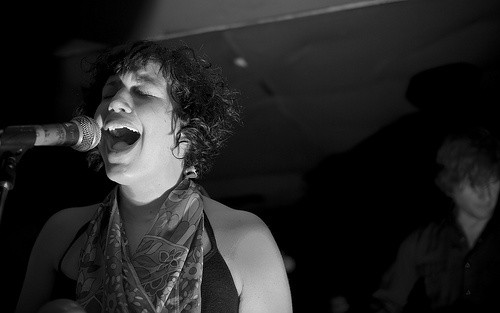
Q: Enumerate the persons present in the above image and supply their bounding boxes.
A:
[16,36,292,313]
[367,126,500,313]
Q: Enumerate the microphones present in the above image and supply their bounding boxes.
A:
[0,115,102,152]
